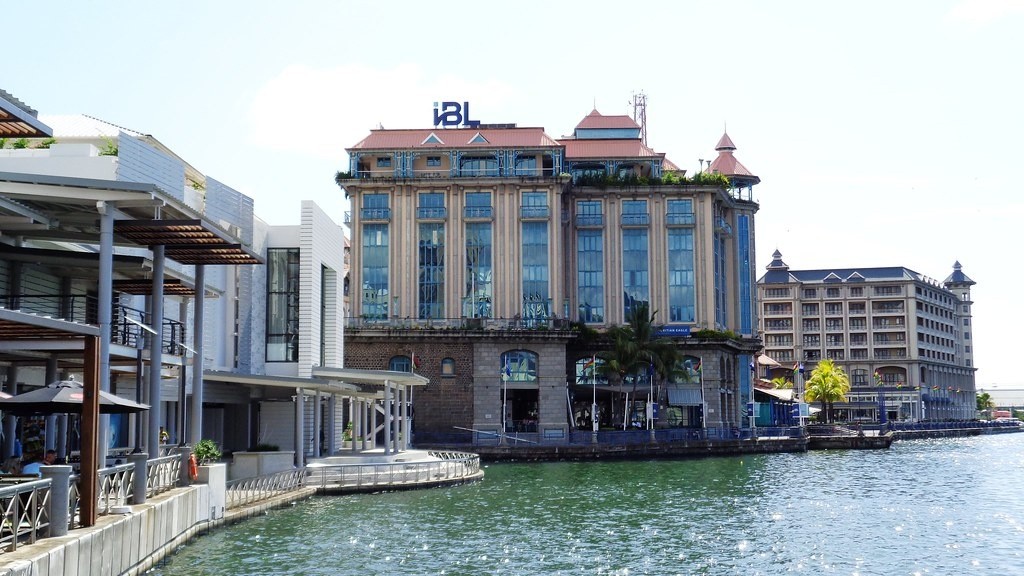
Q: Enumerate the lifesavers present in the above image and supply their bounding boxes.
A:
[189,453,199,480]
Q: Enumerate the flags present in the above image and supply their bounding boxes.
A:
[648,361,655,376]
[793,363,807,375]
[581,355,594,372]
[412,354,421,370]
[913,384,961,397]
[750,358,755,372]
[506,358,512,378]
[894,380,903,391]
[874,368,884,389]
[693,359,702,372]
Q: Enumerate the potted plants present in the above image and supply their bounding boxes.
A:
[344,421,375,449]
[390,433,402,449]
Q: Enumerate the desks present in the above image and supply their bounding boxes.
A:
[68,462,80,473]
[106,456,127,466]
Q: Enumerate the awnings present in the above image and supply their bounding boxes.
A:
[0,374,152,418]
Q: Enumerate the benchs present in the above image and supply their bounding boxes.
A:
[0,473,40,522]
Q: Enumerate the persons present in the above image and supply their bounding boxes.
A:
[0,450,66,477]
[160,424,169,445]
